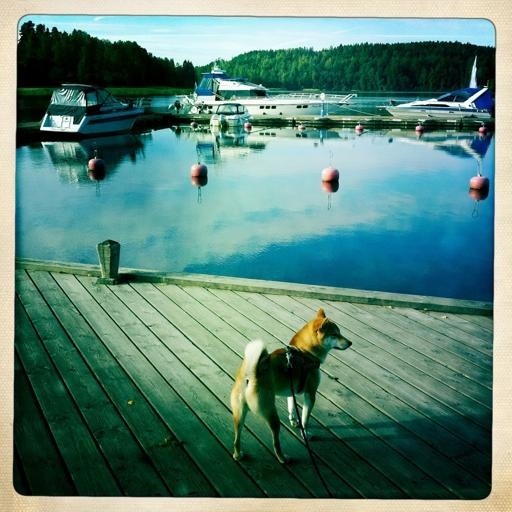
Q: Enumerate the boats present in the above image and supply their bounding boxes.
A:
[167,63,357,119]
[208,102,251,127]
[39,83,145,136]
[385,86,494,119]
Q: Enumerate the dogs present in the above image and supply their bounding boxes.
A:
[228,306,352,466]
